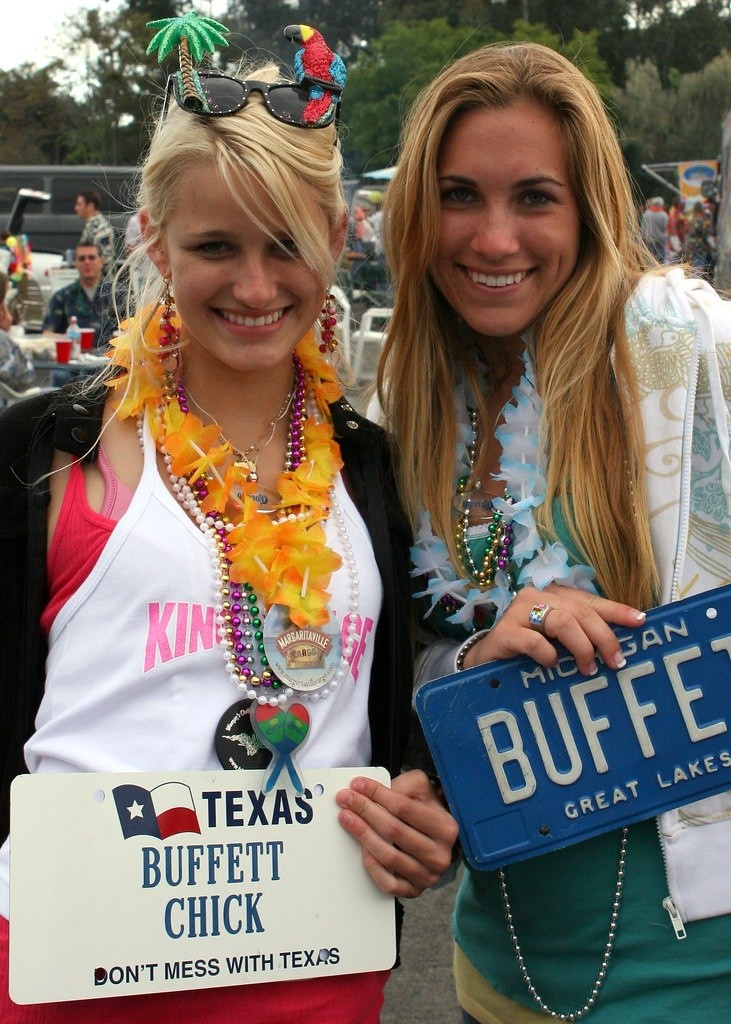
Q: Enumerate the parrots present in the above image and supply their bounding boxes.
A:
[283,23,348,124]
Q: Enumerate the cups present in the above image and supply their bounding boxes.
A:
[80,330,95,353]
[56,340,71,363]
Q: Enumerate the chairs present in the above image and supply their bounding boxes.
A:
[10,269,46,332]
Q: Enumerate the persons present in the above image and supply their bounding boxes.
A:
[0,11,461,1024]
[366,40,730,1024]
[0,191,731,411]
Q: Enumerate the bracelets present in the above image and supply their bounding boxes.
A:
[457,633,487,671]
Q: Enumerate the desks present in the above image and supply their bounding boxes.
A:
[32,347,114,381]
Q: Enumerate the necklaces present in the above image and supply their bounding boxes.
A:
[455,396,517,600]
[136,352,361,705]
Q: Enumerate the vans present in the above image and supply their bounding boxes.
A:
[0,164,146,261]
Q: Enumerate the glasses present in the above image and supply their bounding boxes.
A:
[161,70,342,128]
[75,254,99,262]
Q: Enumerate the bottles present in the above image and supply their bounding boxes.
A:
[66,316,80,359]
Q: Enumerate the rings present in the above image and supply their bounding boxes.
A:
[529,604,554,634]
